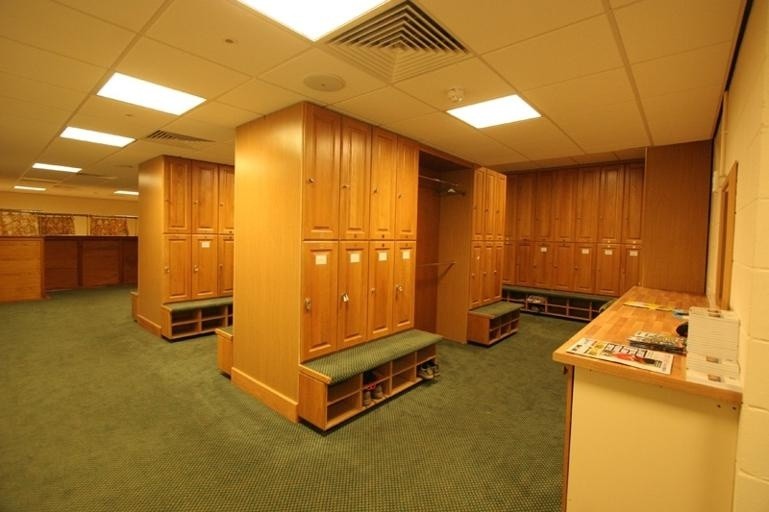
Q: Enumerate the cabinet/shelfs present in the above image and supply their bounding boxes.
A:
[129,154,233,342]
[212,98,442,436]
[434,166,522,348]
[503,161,645,323]
[1,235,138,303]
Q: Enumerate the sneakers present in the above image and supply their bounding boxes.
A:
[532,296,543,304]
[429,362,440,376]
[371,384,383,398]
[527,295,535,303]
[417,364,434,380]
[363,390,371,406]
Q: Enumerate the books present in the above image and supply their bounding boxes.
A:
[626,330,685,353]
[686,306,740,392]
[566,337,675,375]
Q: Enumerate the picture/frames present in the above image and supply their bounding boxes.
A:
[715,160,738,312]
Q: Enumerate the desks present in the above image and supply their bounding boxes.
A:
[550,283,743,512]
